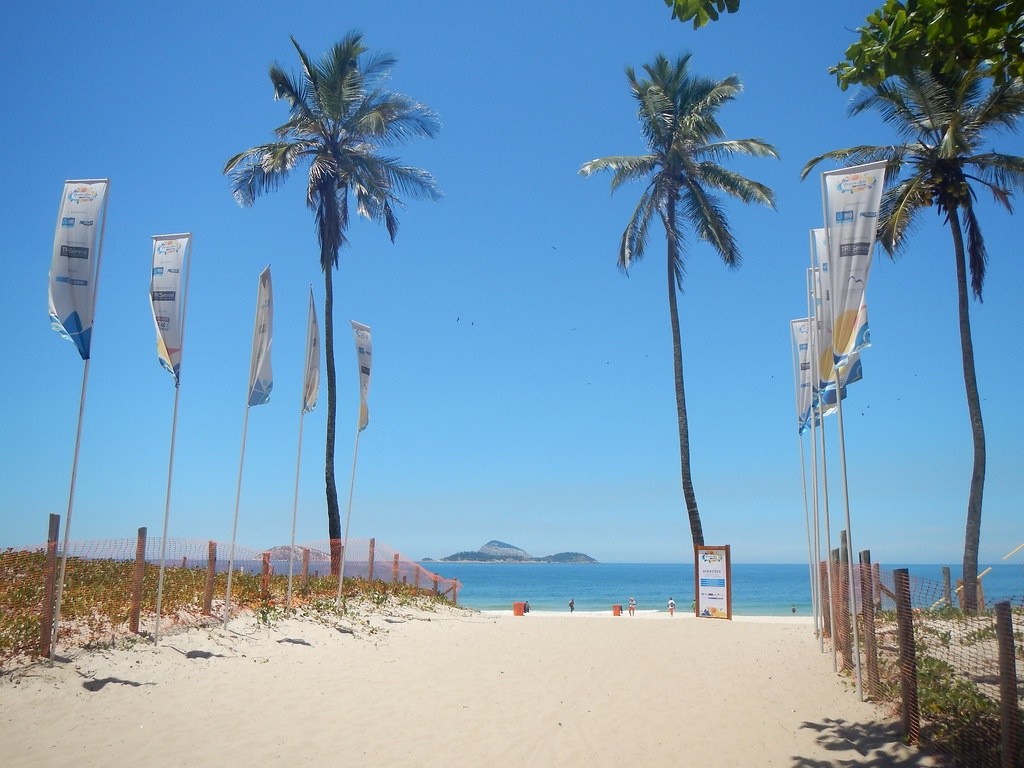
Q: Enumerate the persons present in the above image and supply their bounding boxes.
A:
[792,605,795,613]
[692,600,696,613]
[628,597,637,616]
[569,599,575,612]
[667,597,675,616]
[526,600,529,612]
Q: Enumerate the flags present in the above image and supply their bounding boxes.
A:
[792,227,871,434]
[245,264,274,408]
[826,159,889,371]
[48,178,109,360]
[349,320,373,433]
[149,232,191,388]
[301,287,320,415]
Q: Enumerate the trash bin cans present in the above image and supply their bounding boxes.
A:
[613,605,622,616]
[513,602,525,616]
